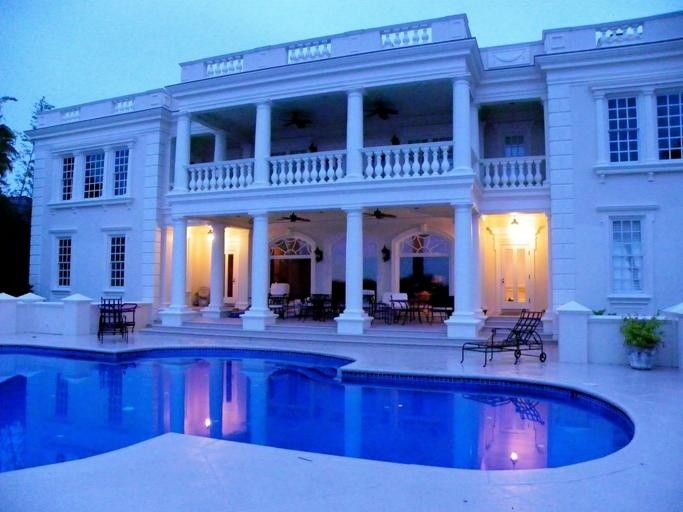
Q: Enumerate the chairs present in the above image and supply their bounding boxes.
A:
[364,283,454,326]
[268,293,344,321]
[98,297,128,344]
[460,309,546,367]
[461,392,545,425]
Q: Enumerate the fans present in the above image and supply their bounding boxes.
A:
[280,111,313,128]
[362,208,396,219]
[364,101,400,120]
[275,213,311,223]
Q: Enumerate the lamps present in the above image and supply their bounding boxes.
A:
[205,418,210,437]
[309,143,318,152]
[510,453,518,470]
[313,247,323,263]
[381,244,391,263]
[390,135,401,145]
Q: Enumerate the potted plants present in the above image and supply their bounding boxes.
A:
[620,316,666,369]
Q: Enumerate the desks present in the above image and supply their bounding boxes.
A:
[98,303,138,335]
